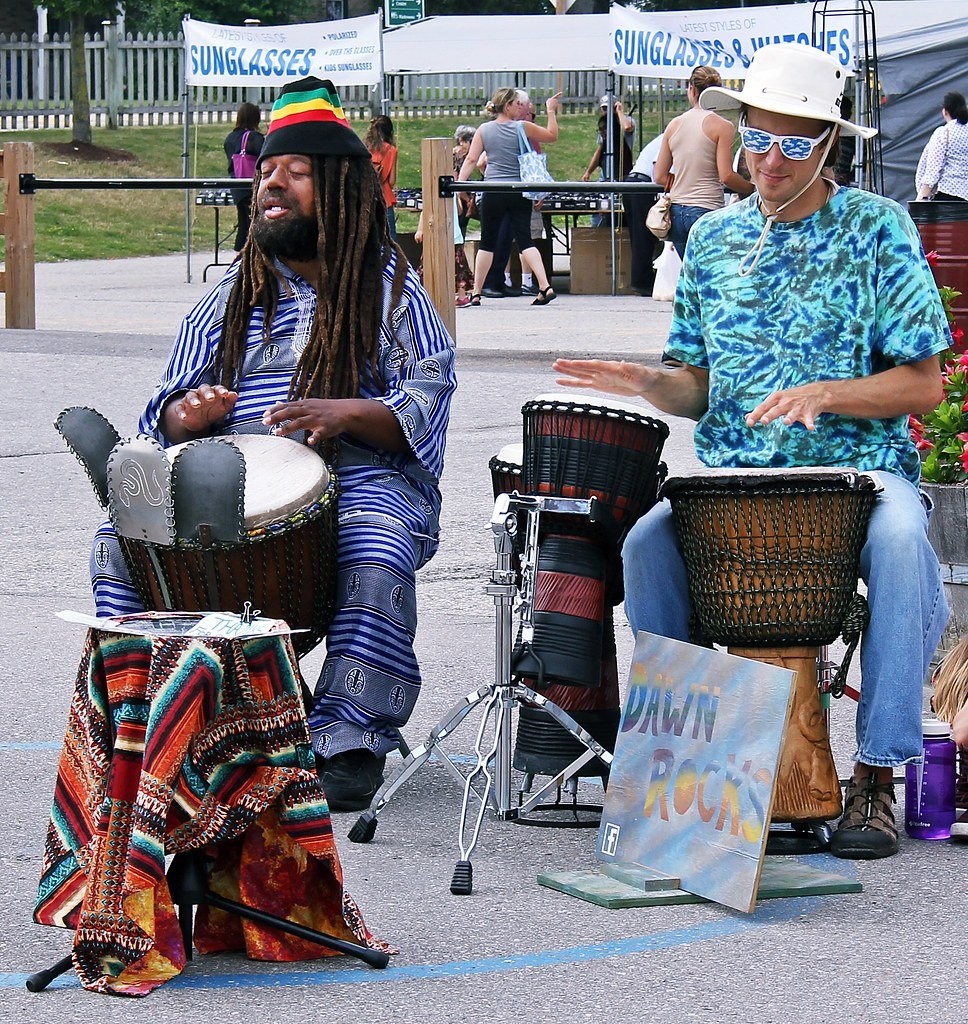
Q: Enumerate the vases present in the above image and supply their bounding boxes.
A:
[915,448,968,690]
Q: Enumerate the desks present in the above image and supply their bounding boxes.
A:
[533,210,623,289]
[196,205,237,283]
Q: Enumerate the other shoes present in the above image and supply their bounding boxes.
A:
[481,281,537,297]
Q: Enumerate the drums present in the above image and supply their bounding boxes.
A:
[489,443,670,780]
[662,466,886,822]
[107,430,343,661]
[507,392,673,691]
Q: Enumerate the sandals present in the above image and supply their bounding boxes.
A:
[467,293,481,305]
[530,286,556,305]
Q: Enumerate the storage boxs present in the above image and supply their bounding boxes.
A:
[463,239,480,275]
[569,226,633,296]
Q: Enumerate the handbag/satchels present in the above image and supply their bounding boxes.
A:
[517,123,556,201]
[651,241,682,302]
[646,195,672,240]
[232,132,258,178]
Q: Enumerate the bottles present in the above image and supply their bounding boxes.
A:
[903,718,957,841]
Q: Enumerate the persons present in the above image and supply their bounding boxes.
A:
[582,95,636,228]
[89,76,459,815]
[654,65,755,262]
[361,114,398,244]
[552,41,955,860]
[622,133,675,297]
[915,90,968,201]
[223,102,266,257]
[414,87,563,308]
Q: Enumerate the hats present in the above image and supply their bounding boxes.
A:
[254,76,372,170]
[600,95,618,107]
[699,44,878,139]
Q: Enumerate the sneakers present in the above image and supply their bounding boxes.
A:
[456,297,472,308]
[317,751,386,811]
[830,775,899,859]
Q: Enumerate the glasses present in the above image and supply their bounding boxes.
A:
[737,112,831,161]
[596,127,607,134]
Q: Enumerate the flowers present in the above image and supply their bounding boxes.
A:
[903,245,968,486]
